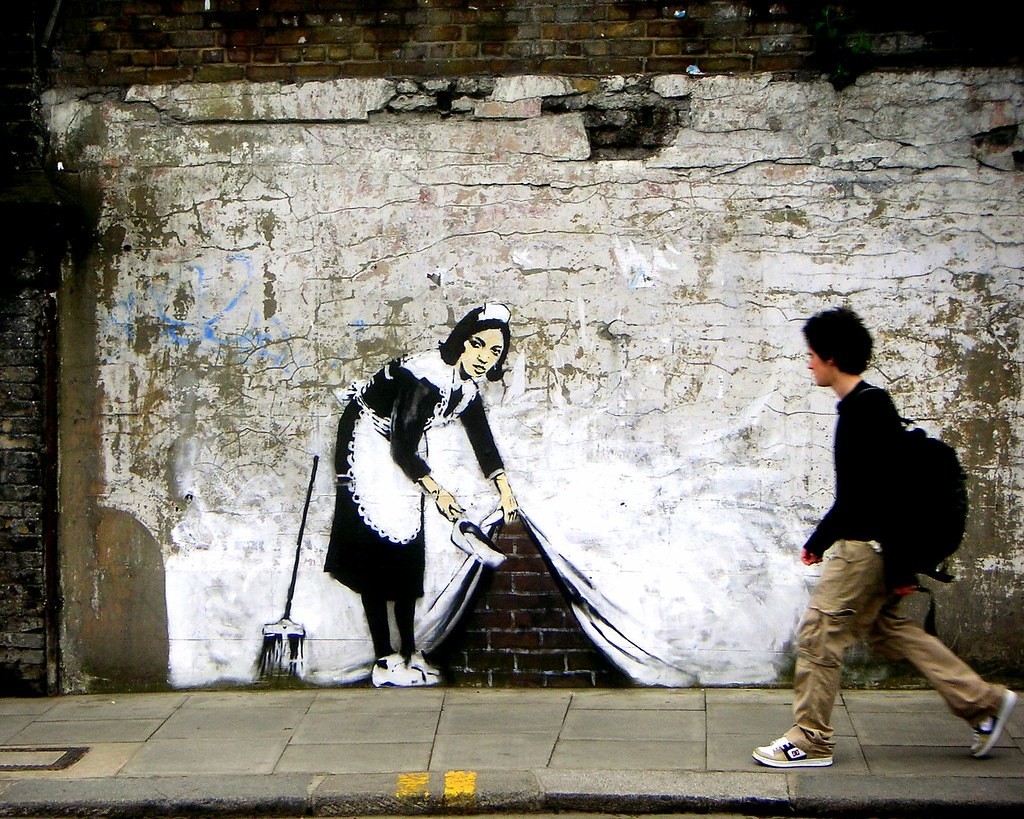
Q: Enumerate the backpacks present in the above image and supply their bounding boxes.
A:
[843,387,967,581]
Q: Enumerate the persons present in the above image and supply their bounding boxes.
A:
[752,305,1018,767]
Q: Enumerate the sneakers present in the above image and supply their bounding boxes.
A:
[970,688,1016,757]
[752,737,833,767]
